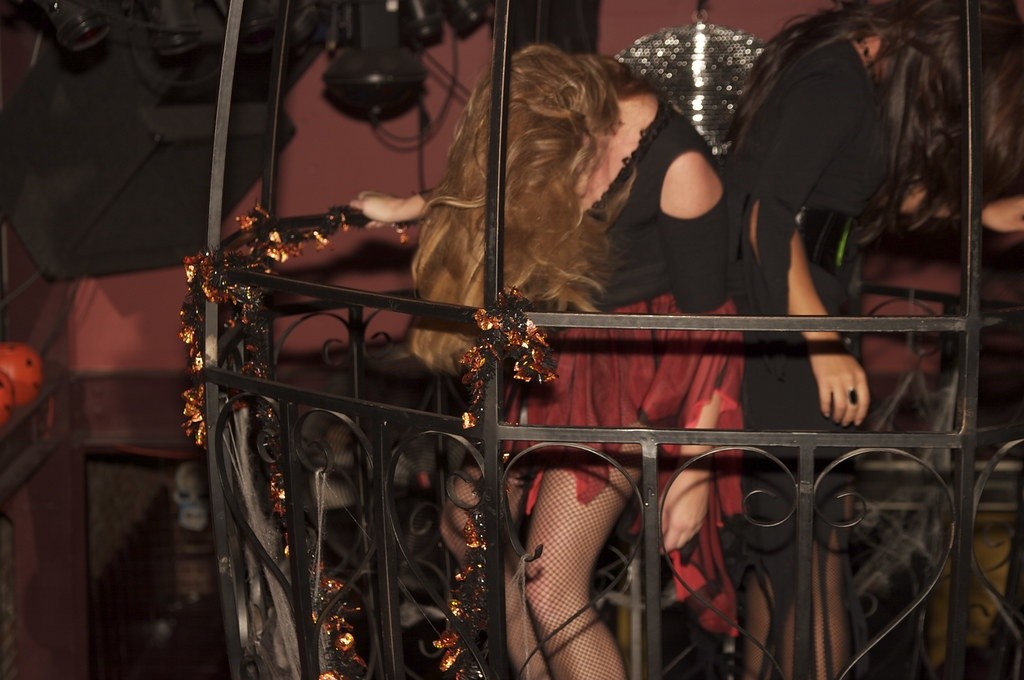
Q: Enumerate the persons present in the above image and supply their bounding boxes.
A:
[349,44,744,680]
[718,0,1024,680]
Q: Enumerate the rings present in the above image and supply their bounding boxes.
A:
[847,388,857,409]
